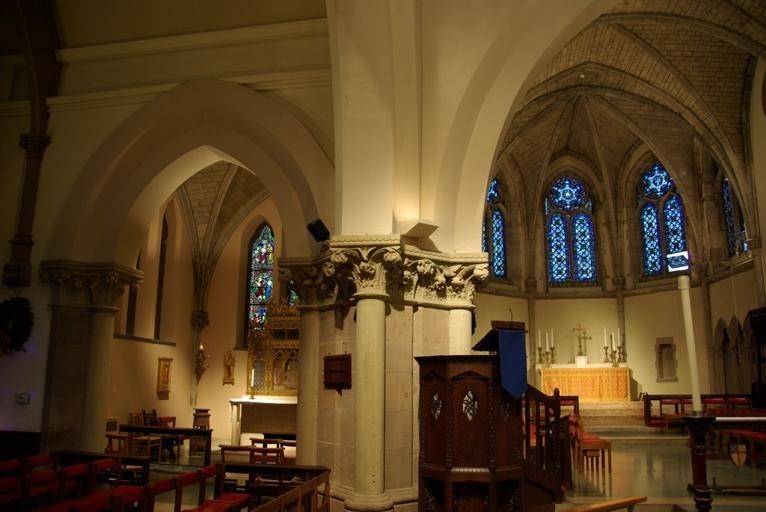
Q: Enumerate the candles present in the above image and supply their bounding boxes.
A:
[537,329,622,351]
[250,367,255,388]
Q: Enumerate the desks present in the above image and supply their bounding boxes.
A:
[227,394,297,450]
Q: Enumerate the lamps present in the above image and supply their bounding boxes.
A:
[306,217,332,241]
[194,341,212,387]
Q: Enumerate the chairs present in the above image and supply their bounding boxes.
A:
[218,437,288,484]
[1,453,254,511]
[104,408,176,463]
[515,392,766,473]
